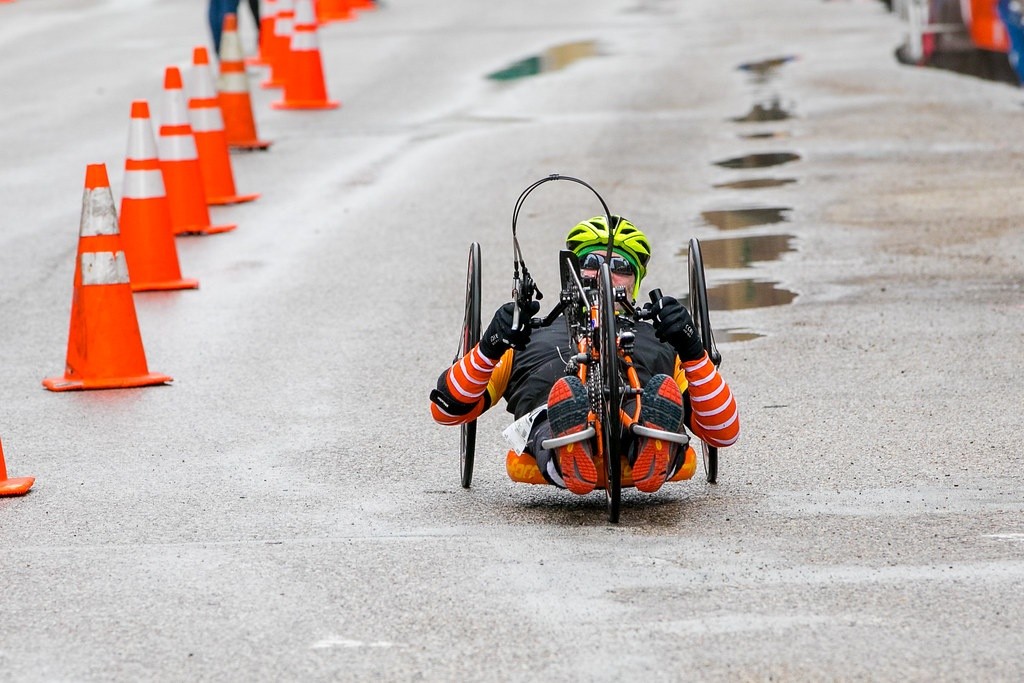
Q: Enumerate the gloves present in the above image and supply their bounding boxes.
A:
[651,295,705,362]
[479,301,540,360]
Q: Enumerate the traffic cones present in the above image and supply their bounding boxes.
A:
[187,46,262,206]
[213,12,274,153]
[235,0,378,90]
[156,66,238,237]
[269,1,342,112]
[0,437,36,498]
[39,162,174,394]
[117,100,199,294]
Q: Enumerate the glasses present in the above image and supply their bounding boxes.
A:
[580,253,634,276]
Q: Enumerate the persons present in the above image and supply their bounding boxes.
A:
[205,0,262,61]
[430,213,741,495]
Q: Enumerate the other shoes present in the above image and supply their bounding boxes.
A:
[632,373,684,493]
[547,374,598,495]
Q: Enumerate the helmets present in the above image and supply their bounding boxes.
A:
[565,216,652,289]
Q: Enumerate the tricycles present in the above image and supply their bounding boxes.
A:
[452,171,723,522]
